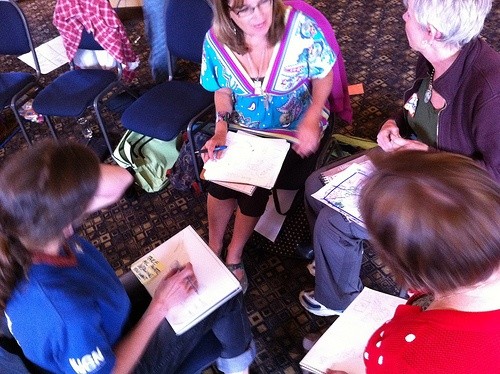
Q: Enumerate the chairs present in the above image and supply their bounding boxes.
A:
[281,0,353,171]
[1,309,221,373]
[121,0,215,192]
[32,0,140,157]
[0,0,46,151]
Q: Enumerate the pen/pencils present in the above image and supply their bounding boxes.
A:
[194,145,228,155]
[177,266,200,294]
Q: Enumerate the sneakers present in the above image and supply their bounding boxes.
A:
[306,259,316,276]
[299,288,345,316]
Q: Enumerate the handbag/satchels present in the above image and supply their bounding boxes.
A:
[111,129,184,193]
[167,121,214,191]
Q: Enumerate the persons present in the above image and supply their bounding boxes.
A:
[199,0,336,291]
[0,140,255,374]
[299,0,499,316]
[326,151,500,374]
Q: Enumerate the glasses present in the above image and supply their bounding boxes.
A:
[226,0,271,17]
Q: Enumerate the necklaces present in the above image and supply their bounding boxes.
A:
[247,46,265,95]
[424,69,435,103]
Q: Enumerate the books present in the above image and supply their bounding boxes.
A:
[319,155,380,221]
[131,226,241,336]
[300,287,407,374]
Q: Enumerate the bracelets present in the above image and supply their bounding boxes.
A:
[215,111,229,123]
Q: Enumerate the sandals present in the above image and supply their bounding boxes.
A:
[224,247,248,294]
